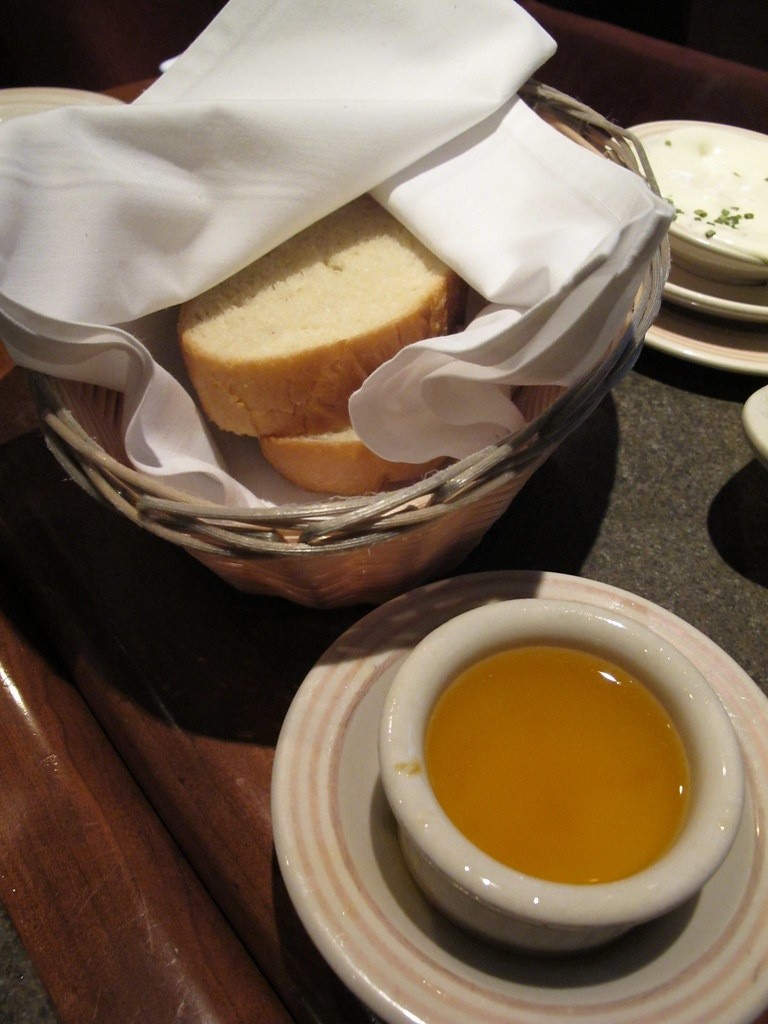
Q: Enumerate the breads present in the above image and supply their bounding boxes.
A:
[176,193,468,438]
[259,413,454,498]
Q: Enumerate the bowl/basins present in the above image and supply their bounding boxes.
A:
[381,599,743,949]
[610,122,768,283]
[740,383,768,467]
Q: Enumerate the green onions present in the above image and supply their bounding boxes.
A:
[662,197,756,240]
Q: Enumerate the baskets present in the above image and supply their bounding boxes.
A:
[23,77,672,611]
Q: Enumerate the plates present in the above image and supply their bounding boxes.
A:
[272,571,768,1024]
[640,263,768,376]
